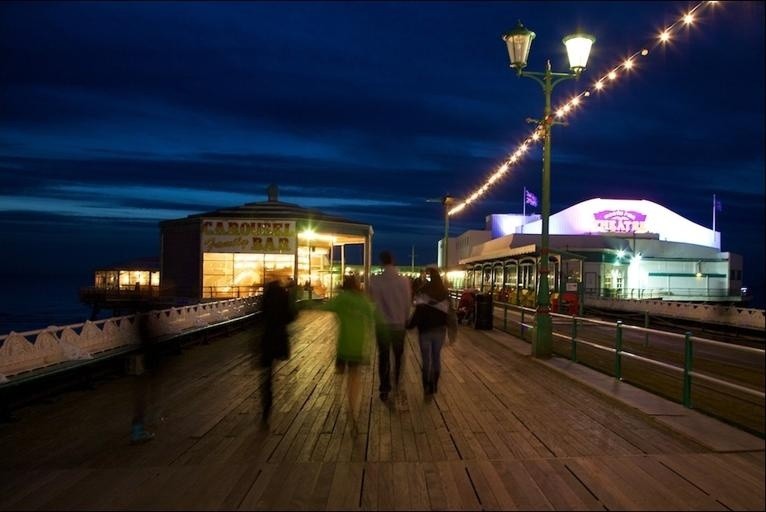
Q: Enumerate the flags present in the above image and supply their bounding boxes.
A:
[525,190,538,207]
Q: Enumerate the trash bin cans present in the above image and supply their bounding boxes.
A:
[473,292,493,330]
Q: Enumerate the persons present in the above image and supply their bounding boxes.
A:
[247,250,459,427]
[125,283,161,441]
